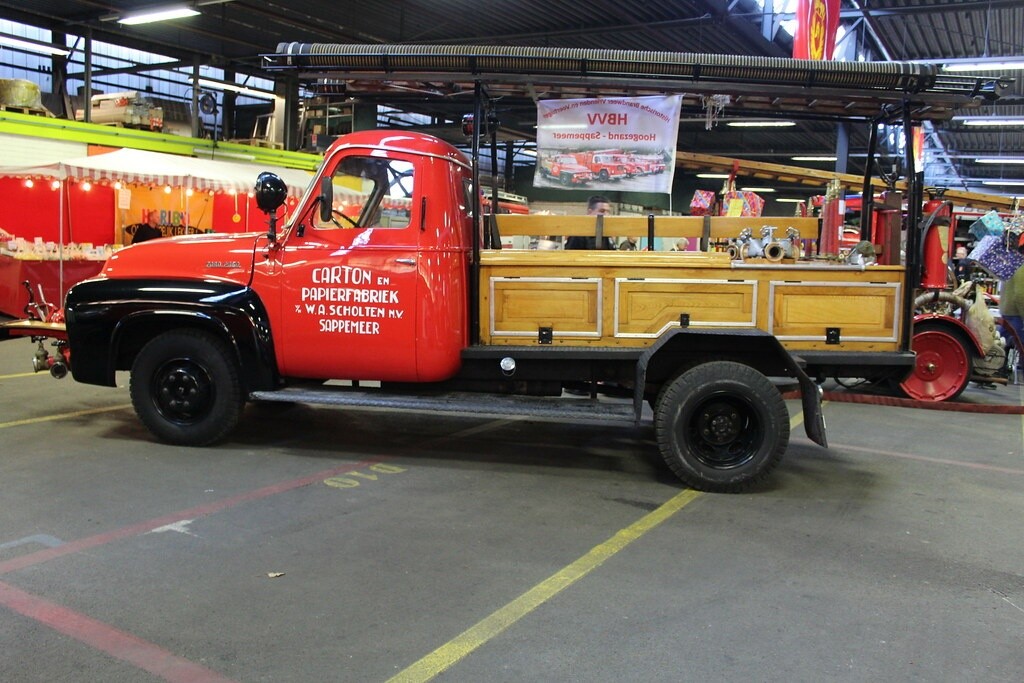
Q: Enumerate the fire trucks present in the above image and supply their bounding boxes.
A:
[569,149,666,182]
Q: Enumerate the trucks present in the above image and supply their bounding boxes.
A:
[539,154,592,185]
[799,194,864,258]
[482,189,529,215]
[64,42,1022,493]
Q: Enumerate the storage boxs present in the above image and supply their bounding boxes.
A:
[967,210,1024,282]
[690,189,766,218]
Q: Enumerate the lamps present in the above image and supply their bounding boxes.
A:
[981,163,1024,186]
[0,30,71,56]
[962,100,1024,126]
[975,126,1024,164]
[187,74,278,100]
[941,1,1024,74]
[117,0,204,26]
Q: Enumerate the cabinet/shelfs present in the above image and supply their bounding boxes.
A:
[299,94,378,149]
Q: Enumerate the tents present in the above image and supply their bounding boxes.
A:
[0,148,411,310]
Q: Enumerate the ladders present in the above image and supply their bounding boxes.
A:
[675,149,1024,214]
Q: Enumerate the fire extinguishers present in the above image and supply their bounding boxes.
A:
[920,187,953,289]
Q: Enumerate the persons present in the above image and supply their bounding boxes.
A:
[669,238,689,253]
[131,211,161,247]
[955,248,967,259]
[619,236,639,250]
[564,195,616,396]
